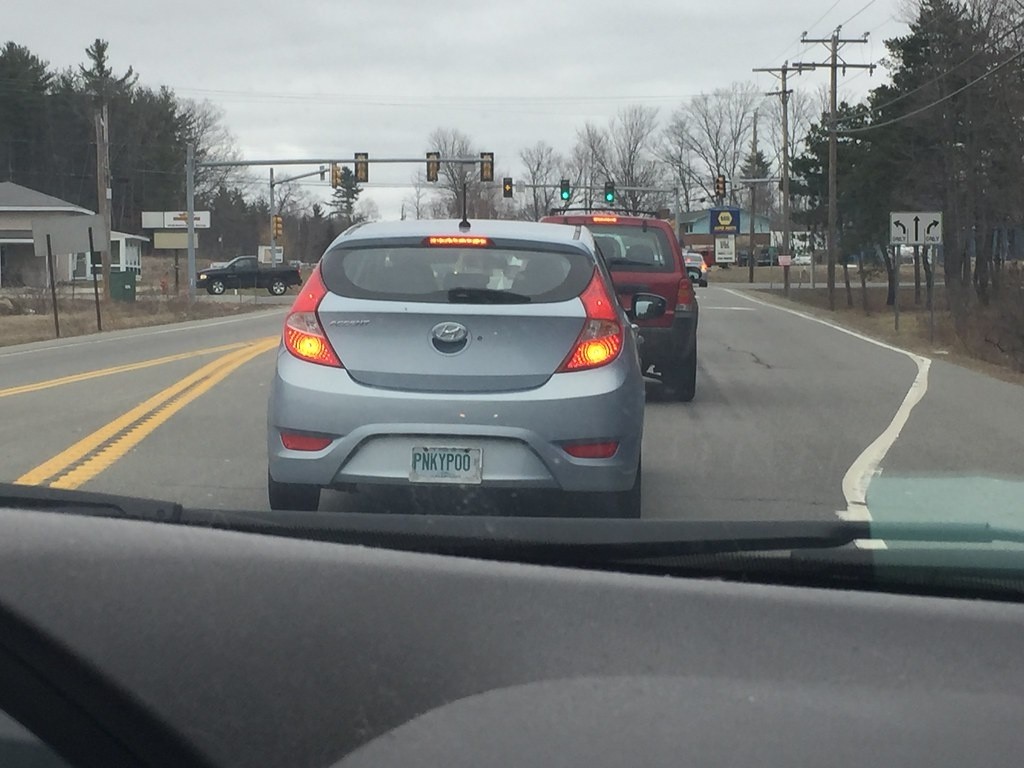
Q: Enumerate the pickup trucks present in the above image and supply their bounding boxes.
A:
[195,255,304,295]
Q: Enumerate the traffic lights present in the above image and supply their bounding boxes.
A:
[605,181,614,202]
[716,174,726,198]
[503,178,513,198]
[561,179,570,201]
[331,162,341,188]
[273,217,283,238]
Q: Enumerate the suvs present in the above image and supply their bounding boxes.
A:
[537,208,704,403]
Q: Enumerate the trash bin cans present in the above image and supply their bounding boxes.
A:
[110,271,136,303]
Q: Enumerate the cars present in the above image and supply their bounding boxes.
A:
[267,219,646,521]
[682,252,708,288]
